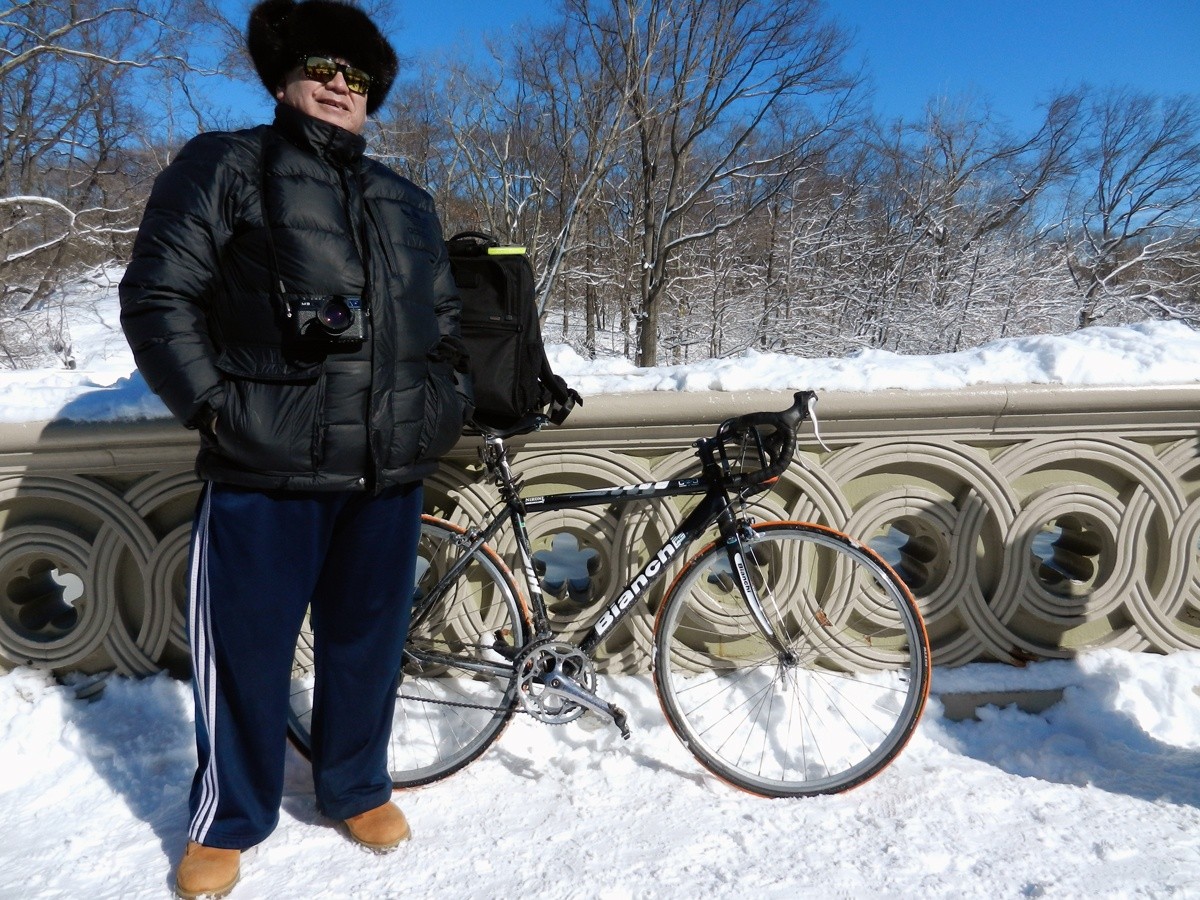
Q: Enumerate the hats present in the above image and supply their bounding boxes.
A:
[246,1,400,116]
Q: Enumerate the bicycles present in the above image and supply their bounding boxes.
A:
[287,390,932,800]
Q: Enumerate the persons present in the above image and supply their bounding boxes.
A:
[120,1,476,900]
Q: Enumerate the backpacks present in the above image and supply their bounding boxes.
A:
[443,227,578,438]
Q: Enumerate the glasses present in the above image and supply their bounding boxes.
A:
[296,52,377,95]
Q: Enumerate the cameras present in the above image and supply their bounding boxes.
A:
[285,295,368,345]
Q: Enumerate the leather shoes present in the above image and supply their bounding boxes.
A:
[341,799,411,849]
[174,839,243,900]
